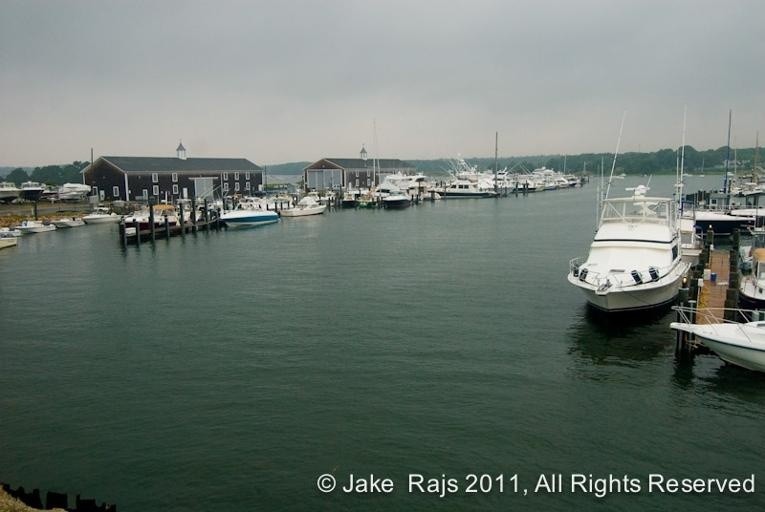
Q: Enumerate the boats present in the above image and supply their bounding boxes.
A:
[116,129,592,235]
[0,179,122,250]
[563,104,764,376]
